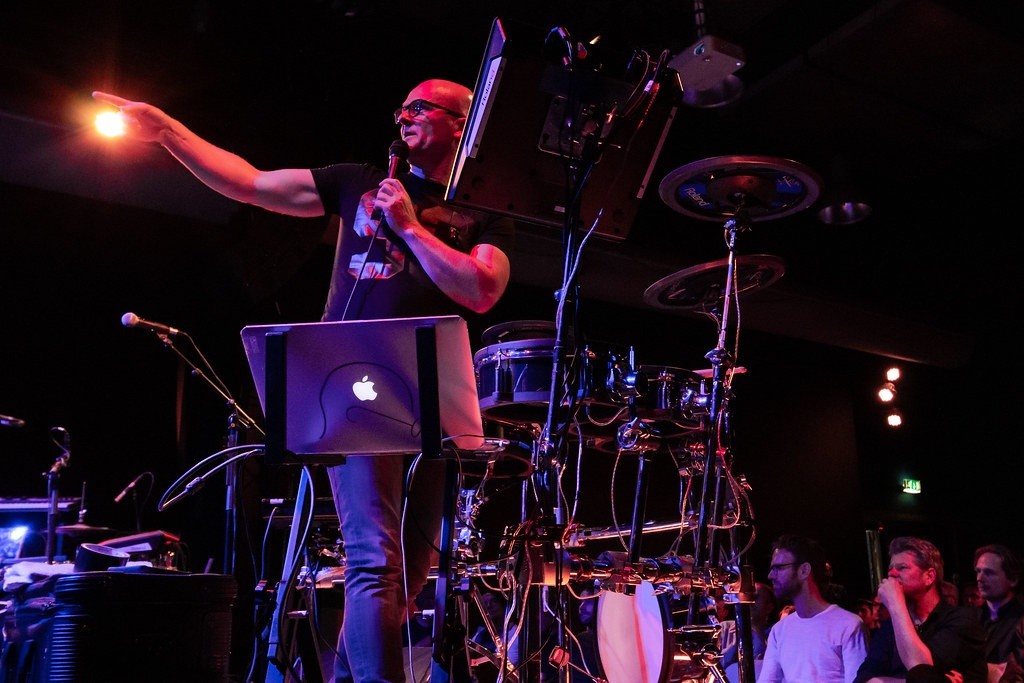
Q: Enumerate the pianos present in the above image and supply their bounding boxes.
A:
[261,494,456,683]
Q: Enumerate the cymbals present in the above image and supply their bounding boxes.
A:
[641,253,789,313]
[660,155,822,226]
[481,320,572,347]
[45,523,111,537]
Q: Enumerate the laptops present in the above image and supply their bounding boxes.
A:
[240,316,499,455]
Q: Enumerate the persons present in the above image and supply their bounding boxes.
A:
[92,79,510,683]
[854,536,1024,683]
[753,534,867,683]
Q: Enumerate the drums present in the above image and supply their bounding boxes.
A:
[568,362,712,461]
[452,473,546,566]
[464,564,676,683]
[455,423,535,479]
[473,337,576,424]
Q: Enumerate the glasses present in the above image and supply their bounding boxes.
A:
[394,98,463,125]
[768,563,800,573]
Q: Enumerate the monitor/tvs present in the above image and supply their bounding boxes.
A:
[443,16,686,253]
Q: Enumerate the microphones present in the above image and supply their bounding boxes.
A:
[63,433,72,453]
[0,415,24,426]
[115,474,143,503]
[381,139,410,220]
[122,313,186,337]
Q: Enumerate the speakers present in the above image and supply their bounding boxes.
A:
[46,570,238,683]
